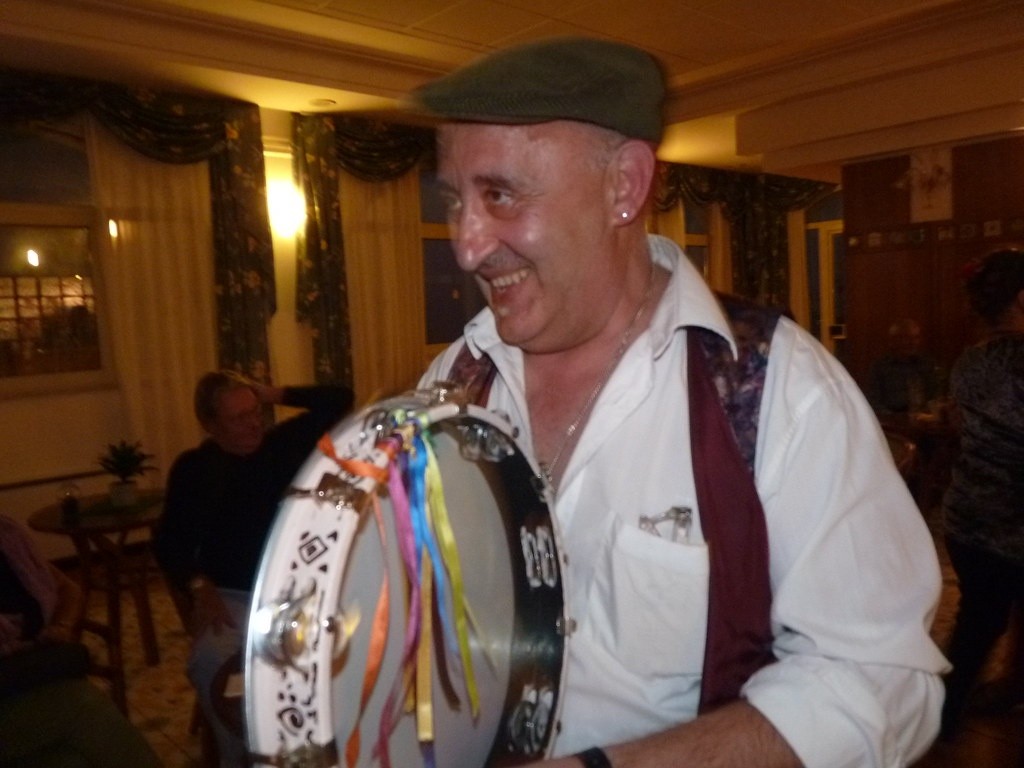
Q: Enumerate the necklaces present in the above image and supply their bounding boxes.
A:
[539,261,656,498]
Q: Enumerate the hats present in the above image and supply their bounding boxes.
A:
[409,36,668,145]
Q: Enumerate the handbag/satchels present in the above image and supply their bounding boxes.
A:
[0,636,93,696]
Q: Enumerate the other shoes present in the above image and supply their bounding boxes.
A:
[941,705,956,737]
[987,672,1024,713]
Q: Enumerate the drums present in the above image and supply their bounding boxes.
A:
[240,388,574,768]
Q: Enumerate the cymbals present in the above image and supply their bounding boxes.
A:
[254,592,352,678]
[521,527,555,583]
[361,408,413,448]
[508,699,551,749]
[458,410,514,461]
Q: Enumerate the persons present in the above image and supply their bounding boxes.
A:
[150,370,355,768]
[728,302,768,353]
[907,242,1024,732]
[413,36,955,768]
[0,514,166,768]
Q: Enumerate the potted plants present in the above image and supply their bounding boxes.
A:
[93,436,161,509]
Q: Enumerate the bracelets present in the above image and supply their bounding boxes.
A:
[186,577,214,593]
[574,747,613,768]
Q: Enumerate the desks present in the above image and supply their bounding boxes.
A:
[25,488,167,717]
